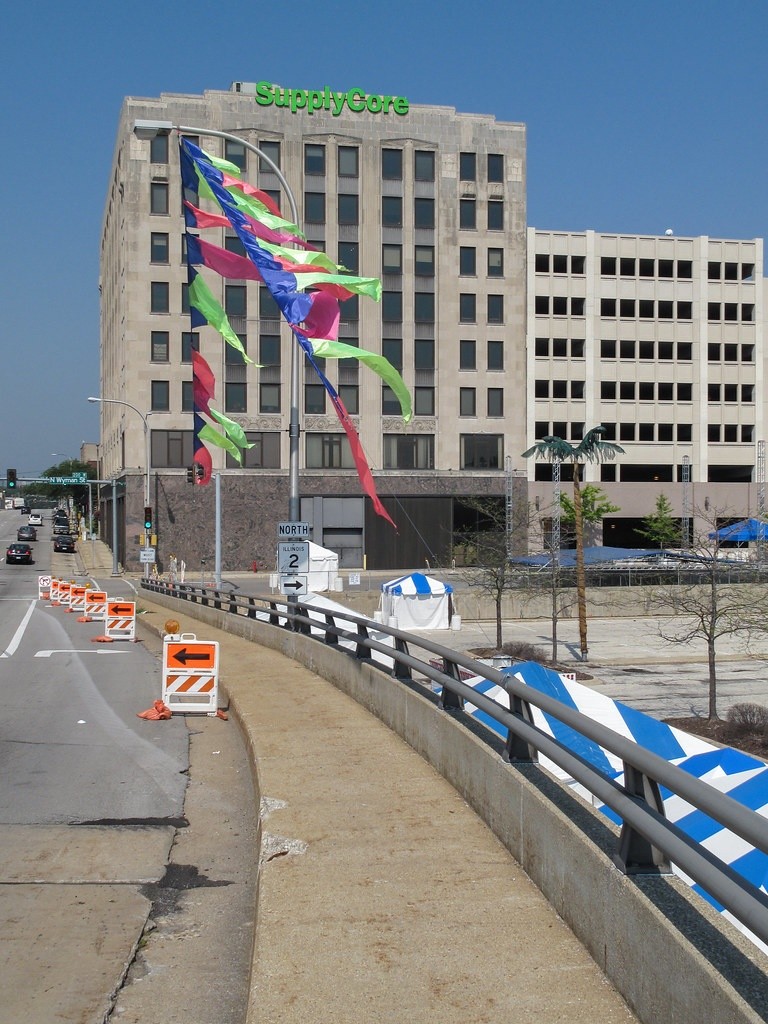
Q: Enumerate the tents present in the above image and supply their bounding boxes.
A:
[276,539,340,592]
[432,660,767,953]
[244,591,396,670]
[379,572,452,631]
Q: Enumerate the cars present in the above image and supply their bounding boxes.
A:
[16,526,37,542]
[52,507,67,523]
[28,513,44,526]
[52,535,77,552]
[21,506,31,514]
[6,543,34,564]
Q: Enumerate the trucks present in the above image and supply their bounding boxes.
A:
[4,497,24,510]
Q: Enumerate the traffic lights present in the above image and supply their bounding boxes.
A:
[144,507,152,528]
[7,469,17,488]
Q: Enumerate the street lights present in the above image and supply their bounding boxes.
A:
[88,397,153,579]
[51,452,74,475]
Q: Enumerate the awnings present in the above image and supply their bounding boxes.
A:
[709,517,767,559]
[503,544,751,568]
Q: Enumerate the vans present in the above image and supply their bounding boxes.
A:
[52,517,70,534]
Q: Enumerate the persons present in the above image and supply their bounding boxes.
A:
[168,551,178,583]
[152,561,160,580]
